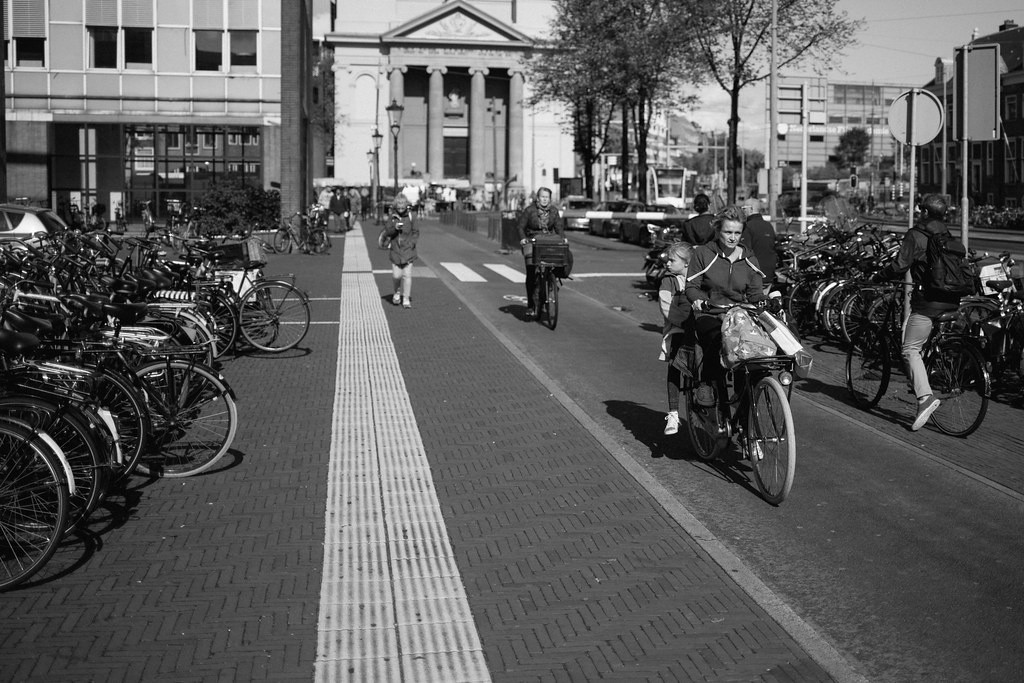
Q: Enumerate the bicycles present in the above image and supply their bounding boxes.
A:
[680,299,798,505]
[57,196,203,235]
[273,202,332,256]
[379,204,392,251]
[0,222,314,592]
[846,273,992,439]
[521,234,570,330]
[645,203,1024,402]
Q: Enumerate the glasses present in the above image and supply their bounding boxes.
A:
[741,206,753,210]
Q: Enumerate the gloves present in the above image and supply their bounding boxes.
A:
[870,273,885,285]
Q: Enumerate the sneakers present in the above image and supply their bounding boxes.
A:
[664,410,682,434]
[737,434,763,459]
[695,383,714,406]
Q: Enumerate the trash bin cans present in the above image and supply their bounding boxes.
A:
[501,210,522,251]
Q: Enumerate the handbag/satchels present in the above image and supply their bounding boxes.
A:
[757,310,804,355]
[552,248,573,279]
[536,232,564,258]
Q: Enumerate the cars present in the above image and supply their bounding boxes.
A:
[556,195,693,243]
[0,203,70,258]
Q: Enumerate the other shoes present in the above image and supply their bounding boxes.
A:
[912,396,940,430]
[392,290,401,304]
[525,307,535,316]
[402,296,411,308]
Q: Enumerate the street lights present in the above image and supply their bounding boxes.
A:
[384,98,405,196]
[367,128,385,228]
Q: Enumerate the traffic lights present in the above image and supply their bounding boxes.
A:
[851,175,858,188]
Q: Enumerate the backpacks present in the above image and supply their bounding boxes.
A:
[907,226,977,295]
[719,306,777,369]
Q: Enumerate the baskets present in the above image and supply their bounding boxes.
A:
[531,243,569,265]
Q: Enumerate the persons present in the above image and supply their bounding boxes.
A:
[517,187,568,316]
[741,198,780,294]
[869,194,961,431]
[383,193,419,308]
[657,242,703,435]
[682,193,715,248]
[686,205,782,461]
[317,180,536,233]
